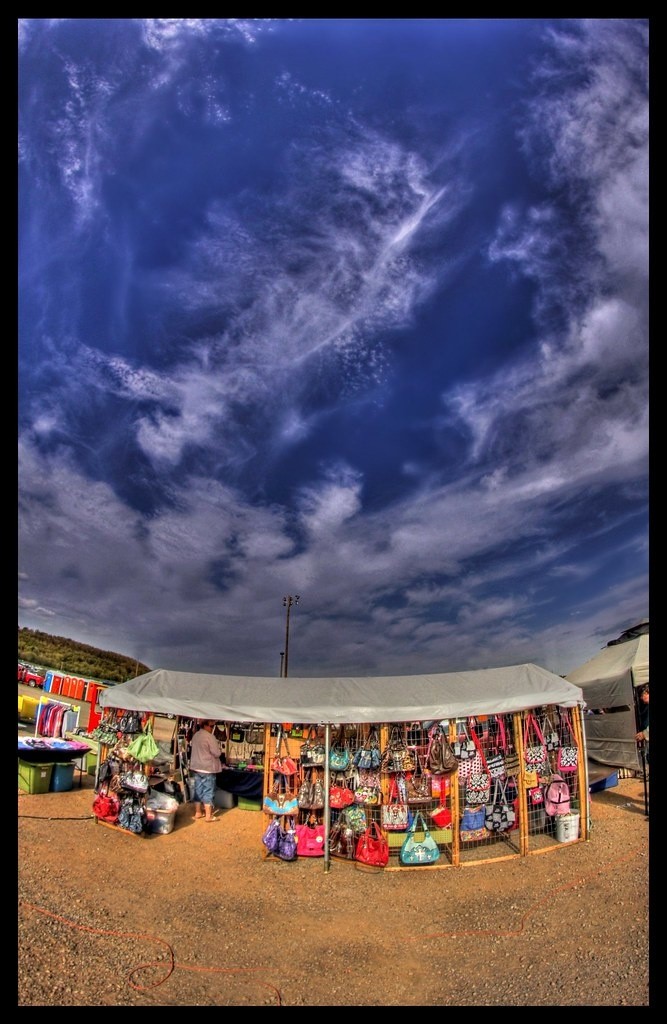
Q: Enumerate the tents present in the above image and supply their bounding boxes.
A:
[563,617,649,786]
[92,663,592,875]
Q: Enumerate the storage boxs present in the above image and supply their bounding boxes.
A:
[214,786,236,809]
[86,752,98,775]
[147,801,180,834]
[73,756,87,771]
[589,772,619,794]
[18,757,55,794]
[50,761,77,791]
[238,796,261,811]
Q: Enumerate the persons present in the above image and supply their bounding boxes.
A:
[190,719,222,823]
[634,682,649,822]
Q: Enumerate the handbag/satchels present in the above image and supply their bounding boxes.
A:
[89,707,160,834]
[171,716,264,768]
[262,712,519,868]
[523,705,580,818]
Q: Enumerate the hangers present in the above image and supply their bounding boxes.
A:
[44,697,76,711]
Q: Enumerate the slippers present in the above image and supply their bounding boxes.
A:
[206,817,220,822]
[195,812,205,819]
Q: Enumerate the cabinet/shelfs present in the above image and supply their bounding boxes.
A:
[97,707,592,873]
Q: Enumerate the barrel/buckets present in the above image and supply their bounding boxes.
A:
[555,809,580,843]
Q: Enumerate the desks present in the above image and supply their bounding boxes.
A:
[18,736,92,788]
[217,767,264,801]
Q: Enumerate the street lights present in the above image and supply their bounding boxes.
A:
[282,595,301,676]
[280,652,284,677]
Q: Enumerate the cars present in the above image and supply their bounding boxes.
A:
[18,664,43,688]
